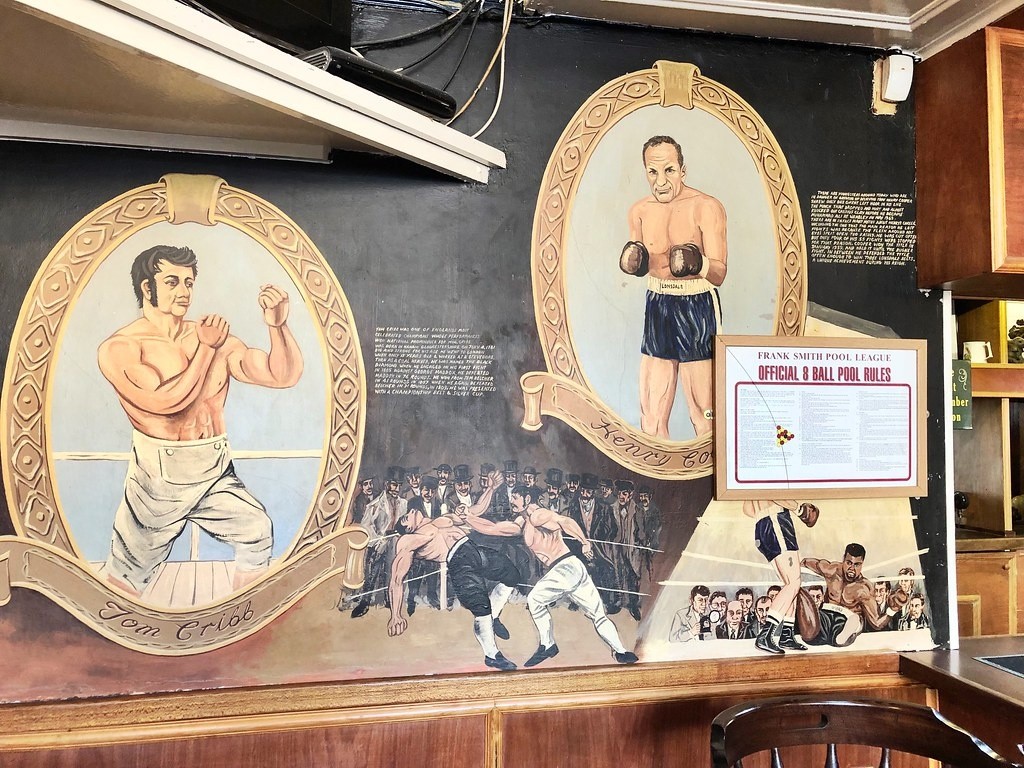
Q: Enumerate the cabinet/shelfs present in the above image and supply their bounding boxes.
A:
[912,29,1024,633]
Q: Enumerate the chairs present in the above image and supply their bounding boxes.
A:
[705,696,1024,768]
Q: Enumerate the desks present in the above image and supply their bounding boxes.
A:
[899,635,1024,768]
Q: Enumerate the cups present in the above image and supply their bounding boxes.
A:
[963,341,993,363]
